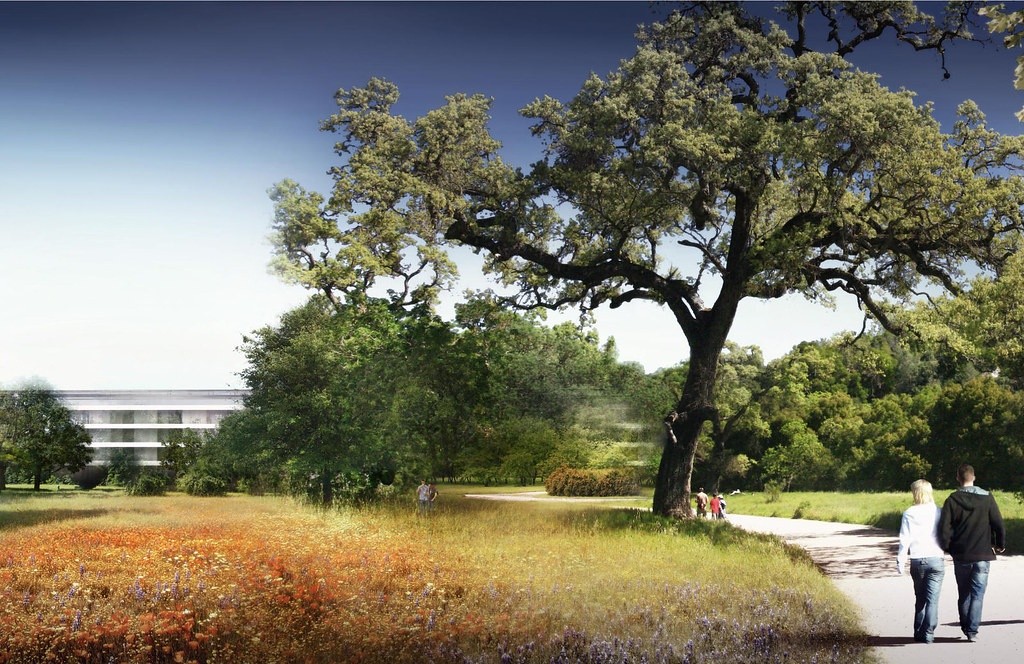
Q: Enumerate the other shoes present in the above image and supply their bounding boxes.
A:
[967,632,980,642]
[914,633,934,644]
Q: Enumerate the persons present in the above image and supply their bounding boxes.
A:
[415,479,430,515]
[938,461,1005,643]
[694,487,708,518]
[710,492,720,521]
[896,478,945,644]
[717,494,726,519]
[427,482,438,514]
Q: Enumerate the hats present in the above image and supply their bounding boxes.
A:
[718,494,723,498]
